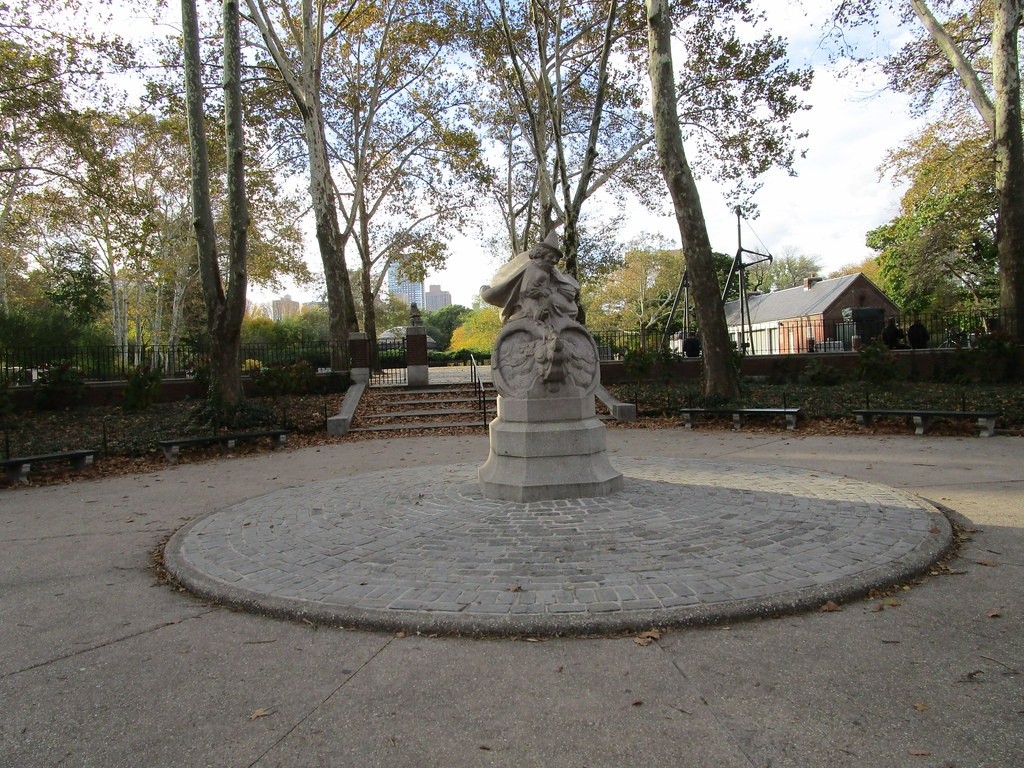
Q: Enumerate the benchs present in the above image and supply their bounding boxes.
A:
[158,430,290,463]
[0,450,99,481]
[680,408,800,430]
[853,409,999,436]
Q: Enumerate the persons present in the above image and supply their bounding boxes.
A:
[480,229,581,321]
